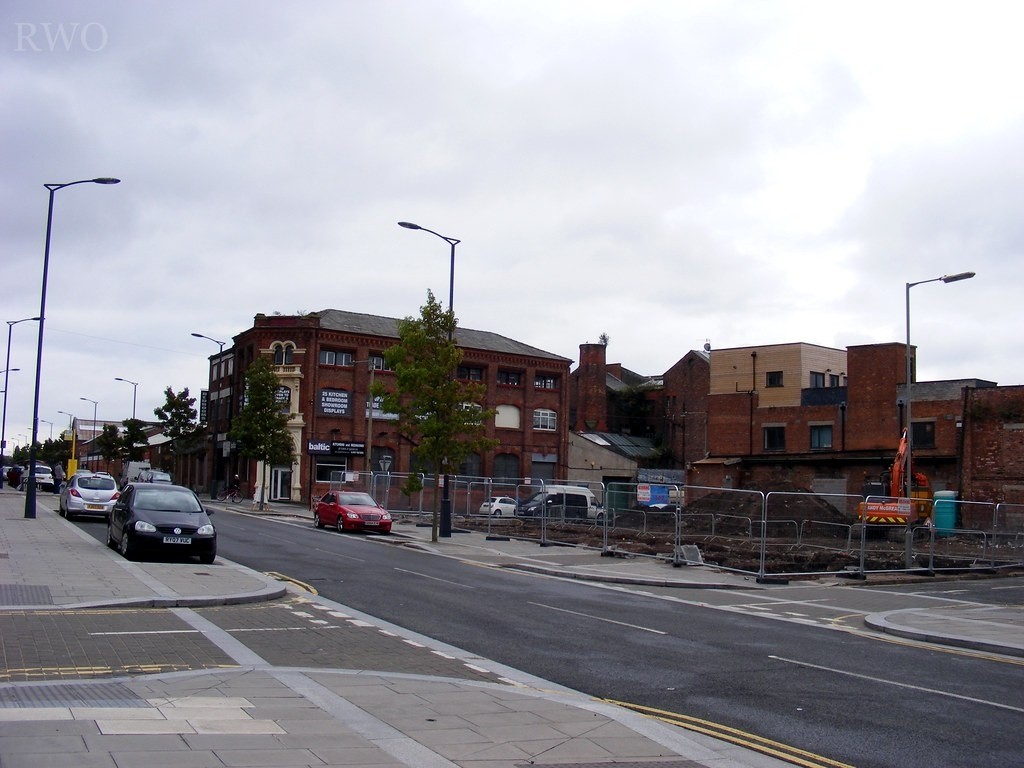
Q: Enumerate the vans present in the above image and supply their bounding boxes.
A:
[539,484,607,524]
[514,492,588,521]
[136,471,173,484]
[119,461,151,491]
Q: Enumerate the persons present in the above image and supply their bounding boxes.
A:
[226,475,241,500]
[52,461,63,495]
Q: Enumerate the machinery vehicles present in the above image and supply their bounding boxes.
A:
[847,426,933,545]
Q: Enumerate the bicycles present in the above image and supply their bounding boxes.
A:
[216,485,245,504]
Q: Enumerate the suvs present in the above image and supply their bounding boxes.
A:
[20,464,54,492]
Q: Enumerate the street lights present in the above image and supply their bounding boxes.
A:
[190,333,227,499]
[57,410,72,430]
[904,270,978,568]
[23,177,122,519]
[40,419,53,439]
[18,434,28,446]
[114,378,138,462]
[8,440,15,452]
[0,315,47,489]
[79,397,98,473]
[397,221,462,538]
[12,438,20,448]
[347,356,376,492]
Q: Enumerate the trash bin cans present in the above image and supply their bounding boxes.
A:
[312,495,321,509]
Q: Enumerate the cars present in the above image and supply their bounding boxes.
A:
[2,465,26,481]
[77,469,93,474]
[59,473,121,524]
[106,482,217,564]
[313,491,392,536]
[479,497,517,519]
